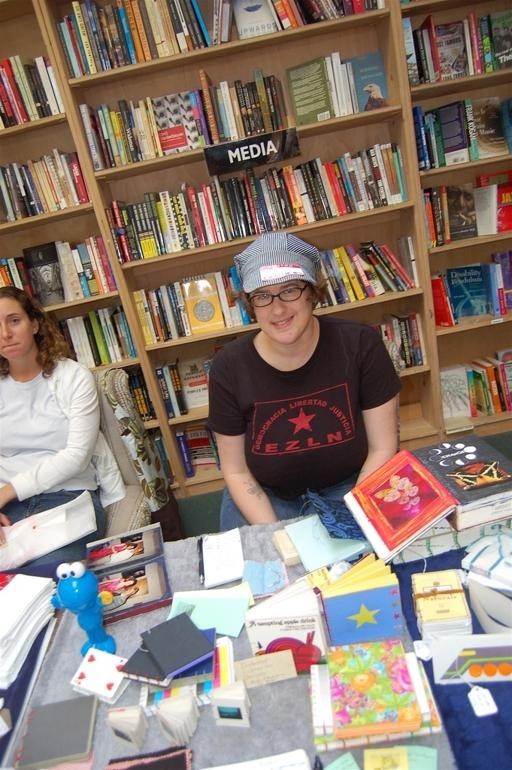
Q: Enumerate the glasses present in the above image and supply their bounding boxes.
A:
[247,283,309,307]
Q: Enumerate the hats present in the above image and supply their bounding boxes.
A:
[233,232,321,293]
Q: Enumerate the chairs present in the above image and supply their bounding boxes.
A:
[90,368,187,542]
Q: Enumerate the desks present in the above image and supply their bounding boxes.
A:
[1,514,512,769]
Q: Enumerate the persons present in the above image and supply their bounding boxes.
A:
[207,232,402,531]
[1,285,109,580]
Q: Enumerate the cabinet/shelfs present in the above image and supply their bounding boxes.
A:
[0,0,512,499]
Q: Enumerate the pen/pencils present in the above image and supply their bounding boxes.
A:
[198,537,204,584]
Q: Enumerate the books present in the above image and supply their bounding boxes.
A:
[401,11,512,245]
[312,237,419,309]
[1,54,118,307]
[431,252,512,420]
[51,1,408,263]
[81,438,511,770]
[54,306,172,487]
[369,311,428,369]
[153,346,221,478]
[132,266,258,345]
